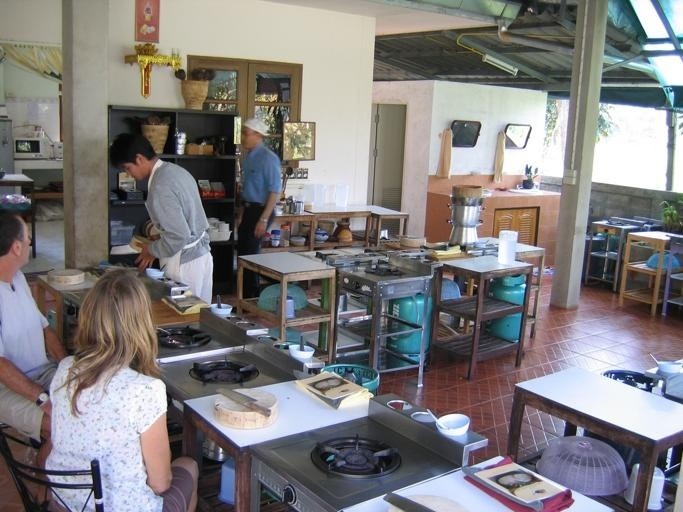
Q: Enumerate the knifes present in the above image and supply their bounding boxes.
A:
[217,384,272,418]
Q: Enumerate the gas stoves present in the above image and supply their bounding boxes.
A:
[153,322,242,364]
[337,259,427,297]
[153,345,305,406]
[247,417,460,512]
[590,216,683,255]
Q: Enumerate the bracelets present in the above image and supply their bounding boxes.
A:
[257,216,270,224]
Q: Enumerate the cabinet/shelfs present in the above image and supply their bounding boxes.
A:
[235,253,335,364]
[105,104,239,296]
[425,257,534,381]
[585,216,683,319]
[236,203,409,288]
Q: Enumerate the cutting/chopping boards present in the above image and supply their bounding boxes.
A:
[45,267,83,285]
[214,387,276,429]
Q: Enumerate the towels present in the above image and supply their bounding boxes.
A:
[492,131,505,182]
[434,129,453,179]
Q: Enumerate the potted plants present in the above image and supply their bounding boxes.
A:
[521,164,538,190]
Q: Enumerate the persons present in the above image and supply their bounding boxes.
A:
[42,268,201,511]
[0,210,74,481]
[235,117,282,299]
[108,131,215,306]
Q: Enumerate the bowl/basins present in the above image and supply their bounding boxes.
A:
[145,268,164,279]
[435,413,470,437]
[656,361,682,373]
[209,303,233,315]
[315,227,329,244]
[288,344,315,358]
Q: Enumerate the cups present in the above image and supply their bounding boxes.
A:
[496,230,518,266]
[205,217,229,232]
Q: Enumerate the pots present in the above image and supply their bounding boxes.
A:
[444,196,486,247]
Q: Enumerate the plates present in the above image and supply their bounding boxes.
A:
[386,400,412,412]
[410,411,437,424]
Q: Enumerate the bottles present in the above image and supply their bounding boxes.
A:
[260,222,289,247]
[334,221,352,243]
[273,198,305,215]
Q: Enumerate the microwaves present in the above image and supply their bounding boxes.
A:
[11,137,49,159]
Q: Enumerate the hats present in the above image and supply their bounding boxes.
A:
[242,118,270,136]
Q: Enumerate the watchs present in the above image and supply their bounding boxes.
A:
[36,390,49,406]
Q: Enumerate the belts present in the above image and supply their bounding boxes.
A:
[243,202,265,207]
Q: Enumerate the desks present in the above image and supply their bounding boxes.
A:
[509,366,683,511]
[13,159,63,202]
[0,173,36,259]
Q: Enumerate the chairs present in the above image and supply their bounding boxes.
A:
[0,433,106,512]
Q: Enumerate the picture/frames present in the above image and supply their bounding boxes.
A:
[281,121,316,161]
